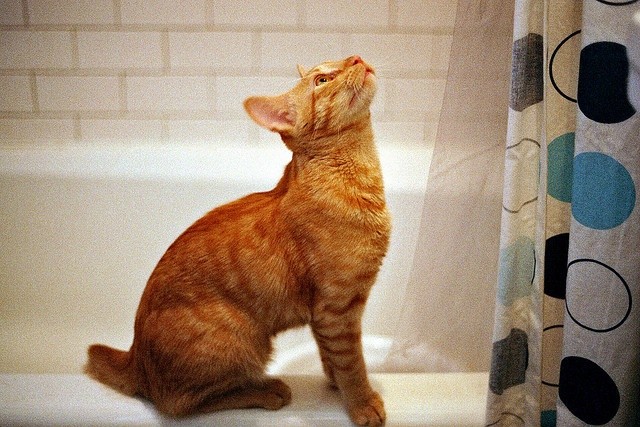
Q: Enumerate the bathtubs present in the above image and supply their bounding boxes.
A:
[1,142,501,424]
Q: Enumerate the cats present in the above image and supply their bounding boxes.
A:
[83,53,394,426]
